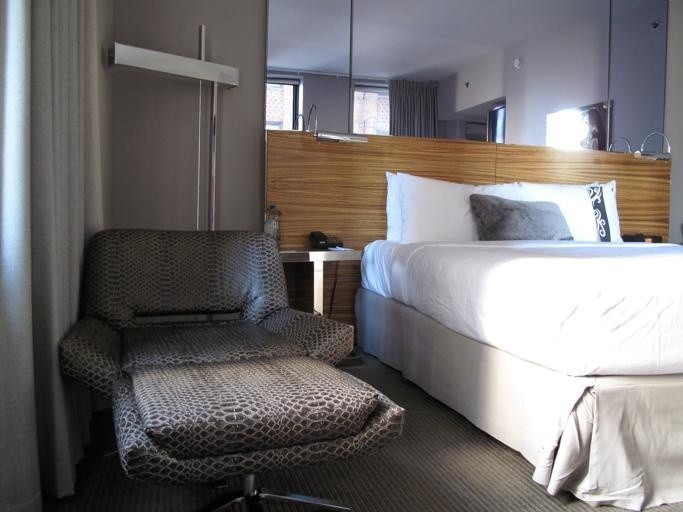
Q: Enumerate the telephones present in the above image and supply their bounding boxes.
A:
[310,231,343,248]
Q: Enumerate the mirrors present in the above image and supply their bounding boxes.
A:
[264,0,668,156]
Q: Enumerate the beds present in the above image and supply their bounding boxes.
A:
[354,237,683,509]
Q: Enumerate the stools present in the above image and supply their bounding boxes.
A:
[111,355,406,505]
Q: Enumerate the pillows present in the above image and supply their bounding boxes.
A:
[385,170,622,242]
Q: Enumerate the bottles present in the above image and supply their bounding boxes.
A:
[265,203,281,243]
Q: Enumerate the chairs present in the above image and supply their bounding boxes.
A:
[59,226,353,383]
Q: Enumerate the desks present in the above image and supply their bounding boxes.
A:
[278,246,361,358]
